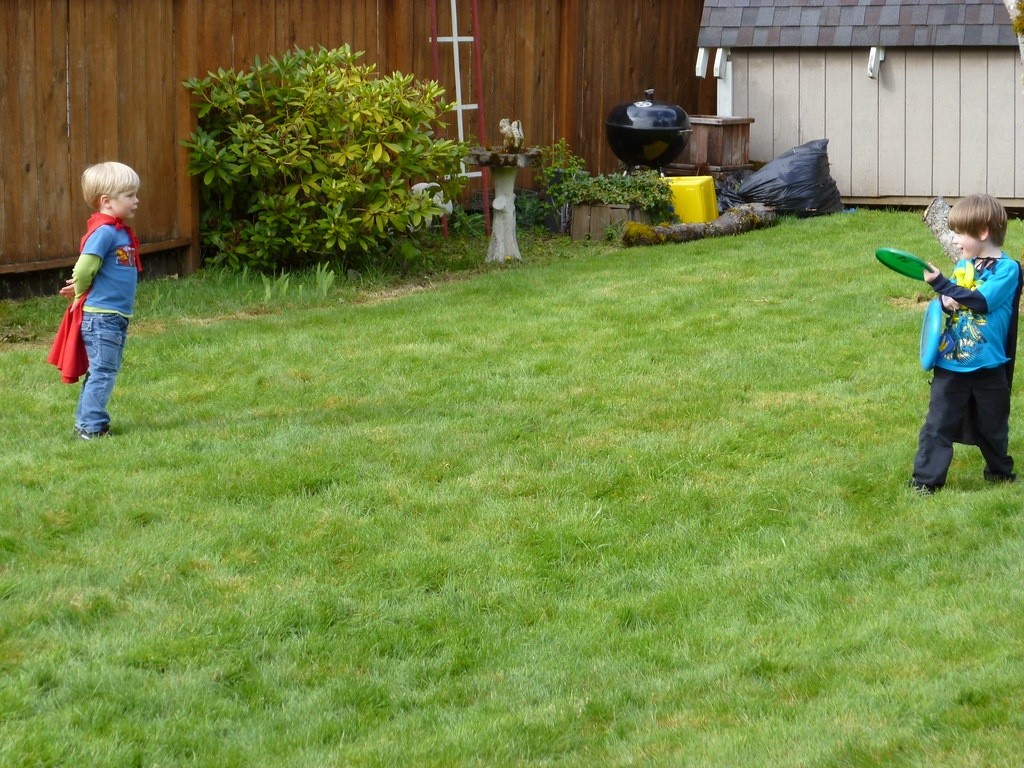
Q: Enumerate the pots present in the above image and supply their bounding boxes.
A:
[605,87,694,169]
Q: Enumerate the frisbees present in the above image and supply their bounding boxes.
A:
[875,245,934,283]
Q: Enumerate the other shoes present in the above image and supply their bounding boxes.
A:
[81,430,112,440]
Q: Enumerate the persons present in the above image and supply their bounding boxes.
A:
[910,195,1024,496]
[60,161,140,441]
